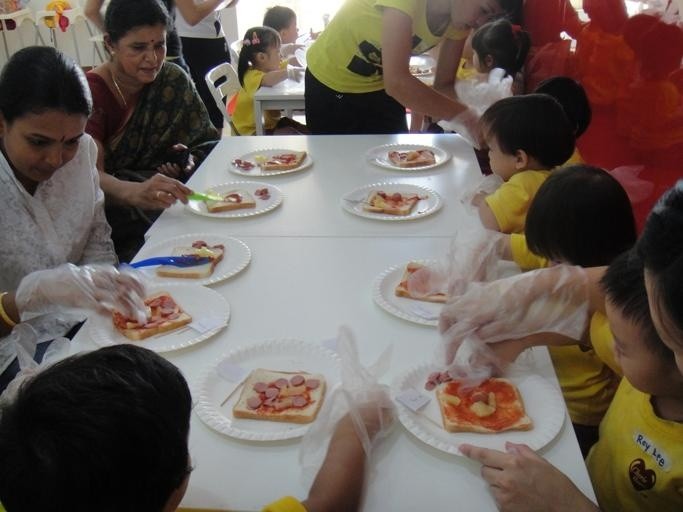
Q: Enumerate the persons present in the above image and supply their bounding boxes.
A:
[534,76,592,170]
[436,178,682,512]
[455,249,682,511]
[0,47,151,381]
[175,0,238,140]
[426,18,531,134]
[470,93,576,236]
[0,344,393,512]
[305,0,524,137]
[232,5,324,136]
[445,164,636,455]
[80,2,219,264]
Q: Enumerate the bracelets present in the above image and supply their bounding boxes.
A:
[0,290,17,328]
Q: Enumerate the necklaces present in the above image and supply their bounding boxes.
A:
[110,67,129,109]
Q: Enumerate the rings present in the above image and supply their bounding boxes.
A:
[156,190,160,199]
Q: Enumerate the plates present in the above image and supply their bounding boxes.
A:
[88,279,231,354]
[363,143,452,172]
[129,233,252,288]
[371,259,475,328]
[191,337,344,441]
[227,146,313,177]
[185,179,283,218]
[341,182,444,221]
[394,357,566,462]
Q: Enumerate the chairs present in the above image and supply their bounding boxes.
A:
[205,38,244,137]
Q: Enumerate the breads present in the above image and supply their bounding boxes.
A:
[363,187,419,216]
[388,147,436,168]
[394,262,453,303]
[205,187,256,211]
[261,149,308,170]
[155,239,226,279]
[110,291,193,340]
[436,377,534,434]
[232,368,327,424]
